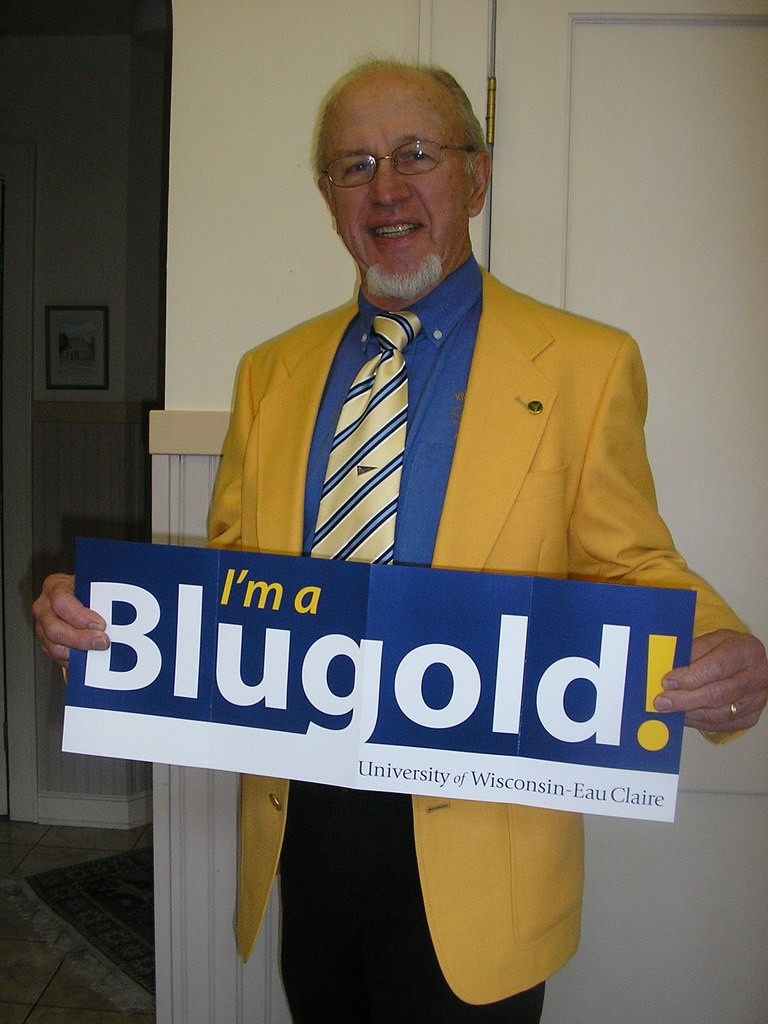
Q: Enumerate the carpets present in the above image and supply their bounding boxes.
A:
[25,847,155,997]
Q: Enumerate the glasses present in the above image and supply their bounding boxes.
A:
[321,139,481,188]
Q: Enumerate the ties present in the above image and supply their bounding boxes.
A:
[309,311,420,566]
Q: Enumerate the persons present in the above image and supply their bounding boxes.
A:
[30,58,768,1024]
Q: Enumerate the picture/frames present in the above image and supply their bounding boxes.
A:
[45,305,110,391]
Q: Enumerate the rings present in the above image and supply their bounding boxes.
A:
[730,703,736,721]
